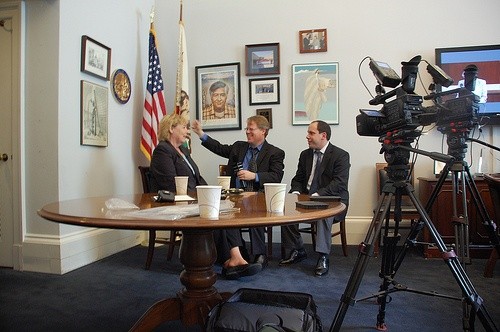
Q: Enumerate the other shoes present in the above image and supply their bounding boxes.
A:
[221,261,262,279]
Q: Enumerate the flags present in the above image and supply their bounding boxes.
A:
[176,20,192,153]
[140,29,167,161]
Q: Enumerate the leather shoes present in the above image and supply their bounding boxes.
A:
[279,248,307,266]
[314,257,330,278]
[253,253,268,271]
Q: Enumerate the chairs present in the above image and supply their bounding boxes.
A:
[299,189,348,259]
[373,162,419,257]
[137,166,184,272]
[219,164,272,260]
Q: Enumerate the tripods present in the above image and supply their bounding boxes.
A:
[387,127,500,281]
[325,161,500,332]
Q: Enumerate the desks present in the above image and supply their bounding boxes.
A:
[35,192,346,332]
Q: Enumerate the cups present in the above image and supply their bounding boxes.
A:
[196,185,222,220]
[174,176,189,195]
[217,176,231,191]
[263,183,287,213]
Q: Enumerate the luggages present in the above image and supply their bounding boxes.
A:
[128,287,323,332]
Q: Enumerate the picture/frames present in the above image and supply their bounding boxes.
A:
[80,80,109,147]
[81,35,110,81]
[291,62,339,125]
[256,108,273,129]
[249,77,280,105]
[110,68,131,104]
[244,42,280,76]
[435,45,500,124]
[299,29,327,54]
[194,62,242,132]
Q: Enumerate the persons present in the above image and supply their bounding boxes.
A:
[279,121,350,278]
[459,71,487,103]
[192,115,285,268]
[150,114,263,278]
[304,33,324,49]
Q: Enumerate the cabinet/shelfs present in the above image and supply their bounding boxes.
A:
[417,177,500,259]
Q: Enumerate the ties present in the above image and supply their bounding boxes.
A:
[243,147,259,192]
[308,151,323,192]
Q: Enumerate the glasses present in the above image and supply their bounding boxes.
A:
[244,127,258,132]
